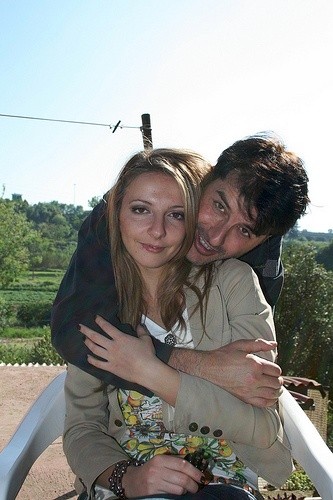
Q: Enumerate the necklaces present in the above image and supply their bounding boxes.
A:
[140,296,185,347]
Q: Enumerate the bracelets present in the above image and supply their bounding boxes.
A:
[107,458,144,500]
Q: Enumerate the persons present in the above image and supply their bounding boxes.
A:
[49,133,315,409]
[61,145,298,500]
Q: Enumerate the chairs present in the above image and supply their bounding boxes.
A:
[2,370,333,500]
